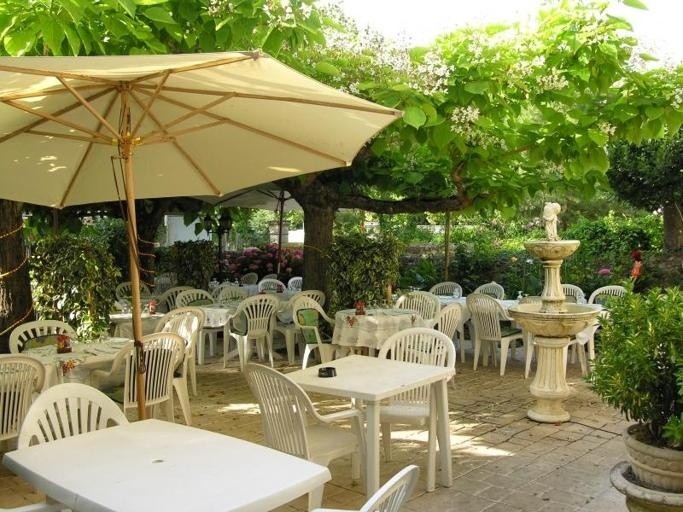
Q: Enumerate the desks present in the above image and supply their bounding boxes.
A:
[2,419,333,511]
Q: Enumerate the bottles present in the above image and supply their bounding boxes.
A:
[516,290,521,301]
[63,336,71,352]
[213,278,217,287]
[122,306,129,317]
[150,297,157,314]
[56,335,64,353]
[112,337,130,343]
[235,278,239,287]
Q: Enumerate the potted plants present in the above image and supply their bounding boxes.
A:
[582,277,682,491]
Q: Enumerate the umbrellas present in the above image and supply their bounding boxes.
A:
[0,47,405,421]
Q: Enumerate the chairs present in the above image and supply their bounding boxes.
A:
[310,465,419,512]
